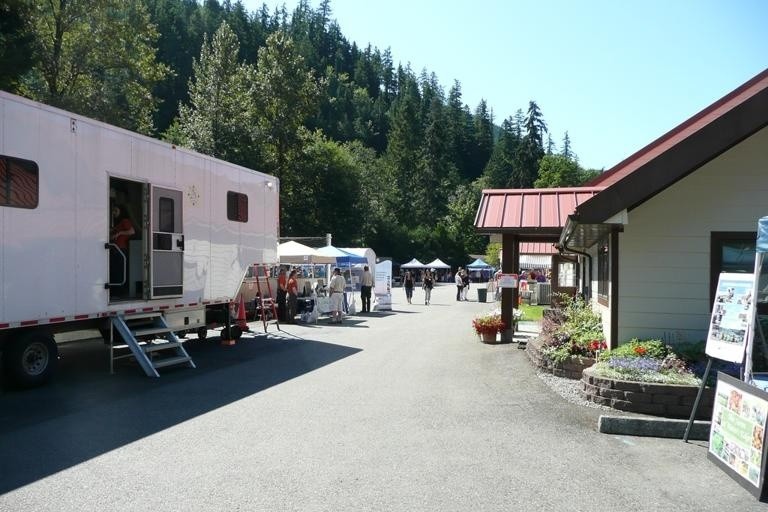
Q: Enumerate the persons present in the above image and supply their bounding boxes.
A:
[109,204,136,273]
[277,268,287,323]
[519,271,527,278]
[286,270,298,323]
[421,269,433,305]
[529,268,536,279]
[327,268,346,324]
[358,266,373,312]
[402,271,414,304]
[454,266,469,300]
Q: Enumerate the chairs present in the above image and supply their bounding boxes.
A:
[249,291,348,325]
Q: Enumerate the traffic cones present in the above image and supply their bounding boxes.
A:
[235,292,249,331]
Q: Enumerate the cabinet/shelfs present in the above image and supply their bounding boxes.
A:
[128,240,142,299]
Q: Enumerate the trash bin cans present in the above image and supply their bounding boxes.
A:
[478,289,487,302]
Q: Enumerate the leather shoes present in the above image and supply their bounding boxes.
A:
[328,320,342,324]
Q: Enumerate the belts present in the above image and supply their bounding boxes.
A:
[334,291,344,293]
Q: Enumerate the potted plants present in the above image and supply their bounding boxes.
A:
[471,307,522,343]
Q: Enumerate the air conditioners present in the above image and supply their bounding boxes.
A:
[537,281,552,305]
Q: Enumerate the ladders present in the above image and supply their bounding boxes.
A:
[255,265,280,332]
